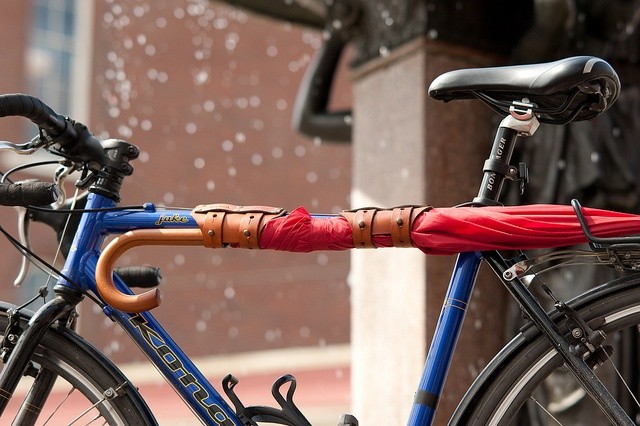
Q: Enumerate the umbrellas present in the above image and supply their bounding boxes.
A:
[96,204,636,313]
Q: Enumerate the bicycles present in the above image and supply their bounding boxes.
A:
[0,55,640,426]
